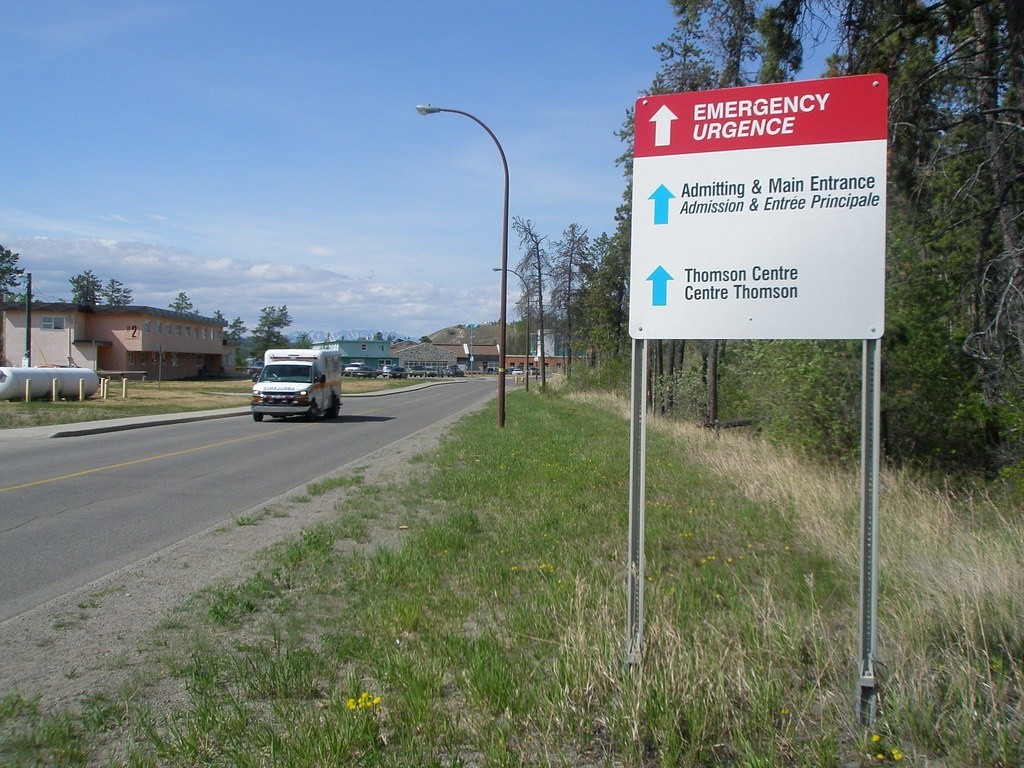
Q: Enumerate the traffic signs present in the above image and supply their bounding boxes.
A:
[630,73,888,340]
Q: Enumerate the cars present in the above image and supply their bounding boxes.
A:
[252,360,264,368]
[484,367,540,375]
[341,362,408,379]
[410,365,464,378]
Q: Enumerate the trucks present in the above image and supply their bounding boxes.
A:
[251,349,343,422]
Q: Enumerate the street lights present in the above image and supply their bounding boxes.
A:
[416,102,509,429]
[18,272,33,369]
[465,323,480,377]
[492,268,530,393]
